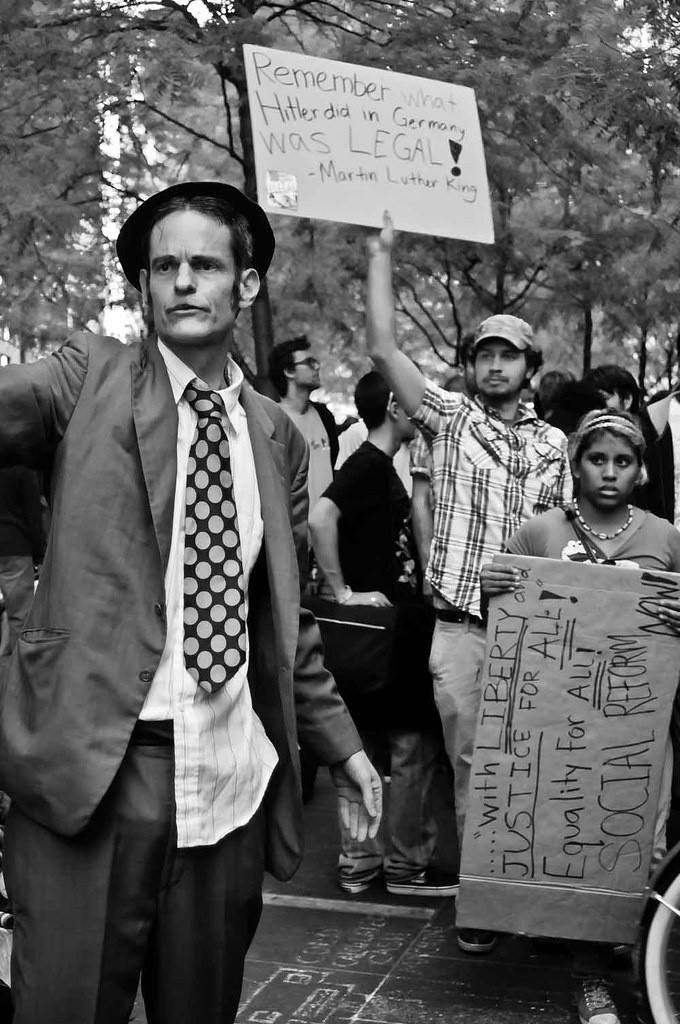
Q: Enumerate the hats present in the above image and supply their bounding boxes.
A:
[115,180,276,299]
[473,313,542,352]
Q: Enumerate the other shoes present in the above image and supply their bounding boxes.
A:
[457,928,500,952]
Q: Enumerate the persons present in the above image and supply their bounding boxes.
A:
[250,333,680,582]
[364,207,579,954]
[307,358,462,897]
[0,180,381,1024]
[479,407,680,1023]
[2,465,48,656]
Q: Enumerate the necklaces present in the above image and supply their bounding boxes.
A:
[572,496,636,541]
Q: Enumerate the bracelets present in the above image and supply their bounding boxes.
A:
[329,585,353,604]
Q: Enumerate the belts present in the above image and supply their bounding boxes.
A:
[132,719,173,745]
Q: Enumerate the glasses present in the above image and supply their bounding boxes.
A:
[291,357,319,367]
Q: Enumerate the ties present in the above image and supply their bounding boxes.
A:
[184,382,248,695]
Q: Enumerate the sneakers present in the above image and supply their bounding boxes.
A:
[574,976,621,1024]
[340,867,461,897]
